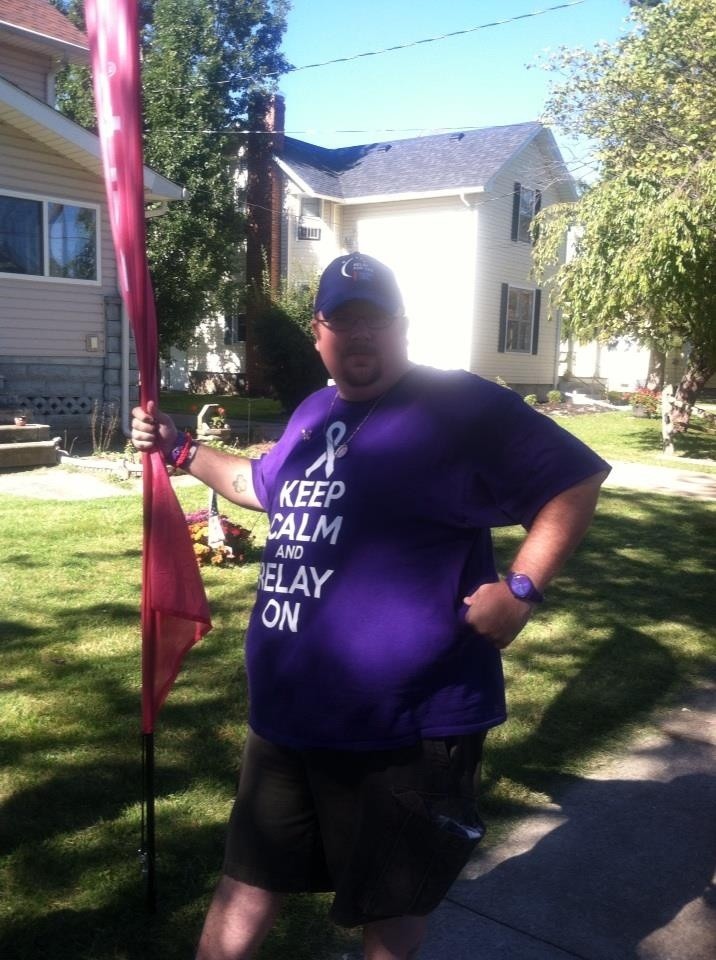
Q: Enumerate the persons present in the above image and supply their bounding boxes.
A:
[118,251,611,960]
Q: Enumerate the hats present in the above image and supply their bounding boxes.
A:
[314,255,404,313]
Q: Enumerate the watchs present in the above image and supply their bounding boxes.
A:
[505,571,544,603]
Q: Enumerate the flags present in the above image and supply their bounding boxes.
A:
[72,0,228,709]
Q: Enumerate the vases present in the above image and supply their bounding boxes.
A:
[632,406,648,417]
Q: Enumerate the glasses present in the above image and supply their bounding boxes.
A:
[316,311,403,331]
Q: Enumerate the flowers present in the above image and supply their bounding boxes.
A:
[629,386,658,410]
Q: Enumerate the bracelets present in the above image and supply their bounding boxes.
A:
[166,424,203,472]
